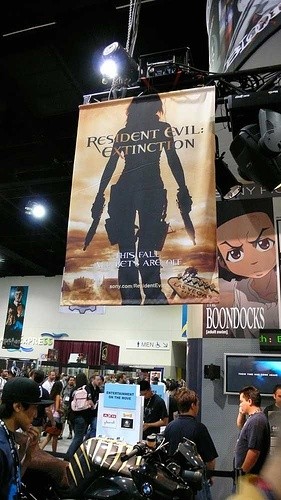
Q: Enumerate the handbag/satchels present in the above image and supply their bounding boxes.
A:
[45,419,63,437]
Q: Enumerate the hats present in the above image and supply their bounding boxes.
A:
[1,378,54,404]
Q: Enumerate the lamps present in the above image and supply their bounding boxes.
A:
[100,42,141,88]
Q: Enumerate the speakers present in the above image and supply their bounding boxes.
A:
[204,365,220,379]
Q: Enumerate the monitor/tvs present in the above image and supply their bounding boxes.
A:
[223,353,281,396]
[268,411,281,437]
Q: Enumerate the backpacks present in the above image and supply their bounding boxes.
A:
[71,385,91,411]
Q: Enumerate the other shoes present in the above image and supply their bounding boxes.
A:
[57,437,62,440]
[67,434,72,439]
